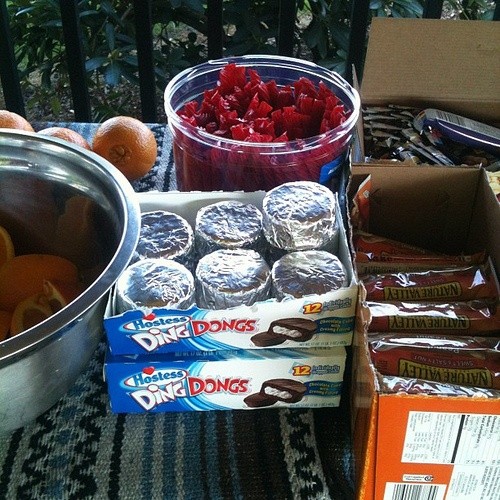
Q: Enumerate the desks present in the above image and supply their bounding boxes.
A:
[2,103,357,499]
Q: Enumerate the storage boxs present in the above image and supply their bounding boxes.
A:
[104,13,499,499]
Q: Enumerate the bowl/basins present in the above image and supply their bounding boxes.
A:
[1,124,142,436]
[164,51,361,190]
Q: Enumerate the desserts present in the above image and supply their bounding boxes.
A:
[249,318,318,347]
[242,379,308,406]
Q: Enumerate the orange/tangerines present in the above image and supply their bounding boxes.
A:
[0,110,156,346]
